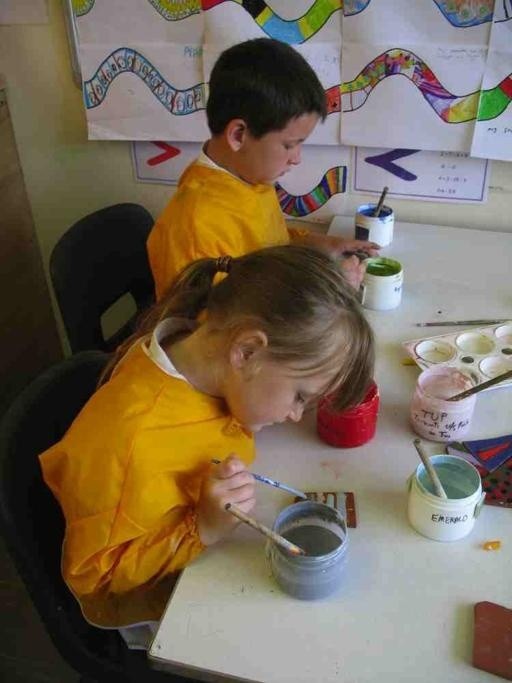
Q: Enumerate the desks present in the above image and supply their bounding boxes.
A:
[147,215,512,683]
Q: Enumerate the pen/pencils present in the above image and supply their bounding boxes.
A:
[374,185,390,215]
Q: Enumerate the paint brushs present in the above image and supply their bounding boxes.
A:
[211,459,312,500]
[415,320,512,327]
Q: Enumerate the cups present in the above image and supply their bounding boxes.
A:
[263,500,351,601]
[358,256,404,310]
[316,376,379,449]
[354,202,396,247]
[409,365,476,441]
[407,454,489,544]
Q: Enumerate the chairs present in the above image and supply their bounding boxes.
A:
[50,203,154,352]
[0,350,137,683]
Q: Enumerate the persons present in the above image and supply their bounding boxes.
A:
[144,37,381,314]
[36,245,377,629]
